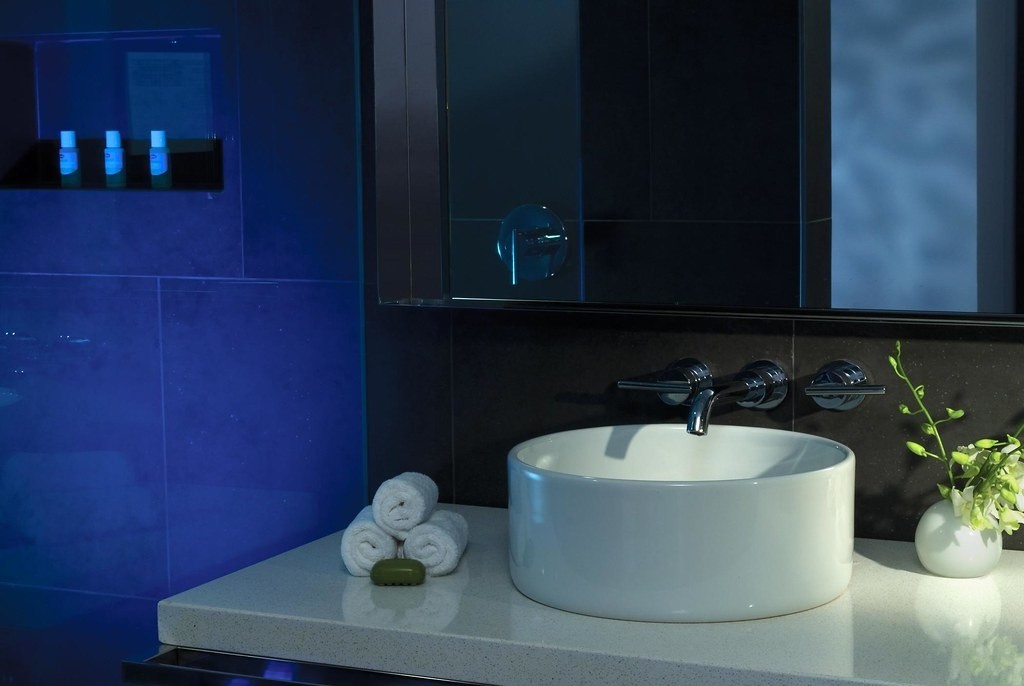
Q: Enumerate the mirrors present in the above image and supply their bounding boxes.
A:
[435,2,1021,318]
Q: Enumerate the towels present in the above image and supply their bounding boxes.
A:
[339,471,469,577]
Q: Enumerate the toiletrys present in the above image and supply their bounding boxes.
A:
[58,129,169,176]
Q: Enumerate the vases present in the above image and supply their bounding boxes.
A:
[914,497,1003,578]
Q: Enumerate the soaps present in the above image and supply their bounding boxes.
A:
[369,558,427,586]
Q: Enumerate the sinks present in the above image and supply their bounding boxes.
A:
[507,423,856,623]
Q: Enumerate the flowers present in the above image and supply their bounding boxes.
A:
[884,340,1023,537]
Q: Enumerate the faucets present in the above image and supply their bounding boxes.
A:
[687,359,789,435]
